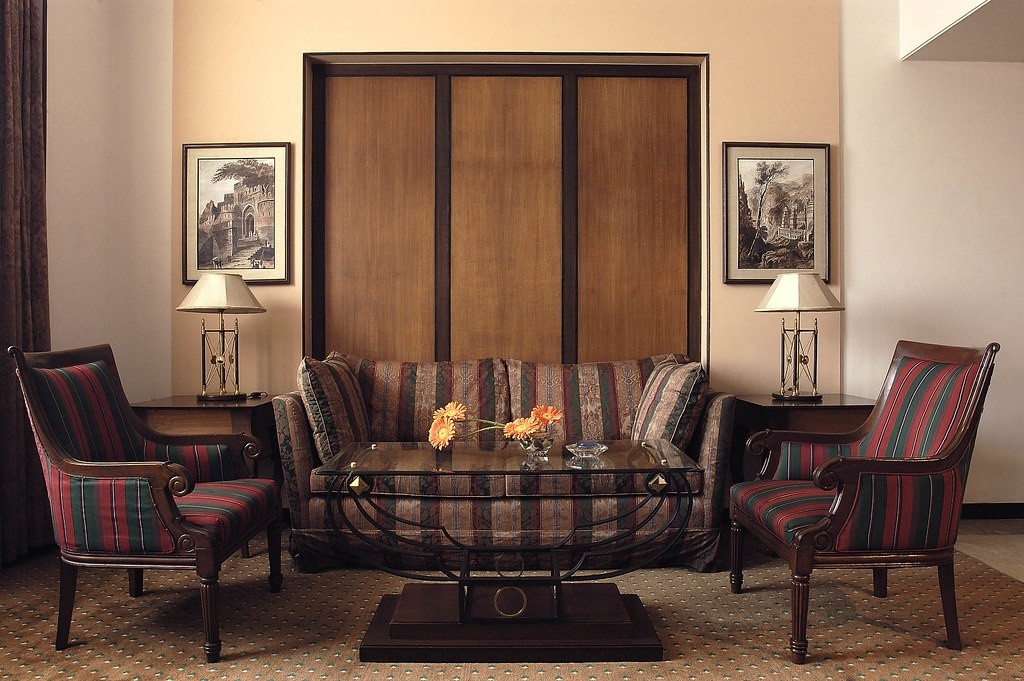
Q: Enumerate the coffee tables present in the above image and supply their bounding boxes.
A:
[315,438,705,660]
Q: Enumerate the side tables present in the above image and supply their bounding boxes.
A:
[734,393,877,480]
[129,393,287,528]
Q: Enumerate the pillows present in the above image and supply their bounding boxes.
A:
[297,349,372,464]
[630,352,709,453]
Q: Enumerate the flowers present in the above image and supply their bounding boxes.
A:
[425,403,565,451]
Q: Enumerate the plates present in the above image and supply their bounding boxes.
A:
[565,441,609,457]
[566,455,604,470]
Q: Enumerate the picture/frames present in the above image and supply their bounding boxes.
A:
[721,141,830,285]
[181,142,290,284]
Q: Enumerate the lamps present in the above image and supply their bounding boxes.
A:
[177,272,269,403]
[754,271,846,401]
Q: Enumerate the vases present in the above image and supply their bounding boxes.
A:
[519,431,557,457]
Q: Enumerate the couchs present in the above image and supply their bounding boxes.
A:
[7,343,284,663]
[273,350,734,575]
[729,339,998,668]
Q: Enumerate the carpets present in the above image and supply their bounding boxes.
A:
[0,550,1023,681]
